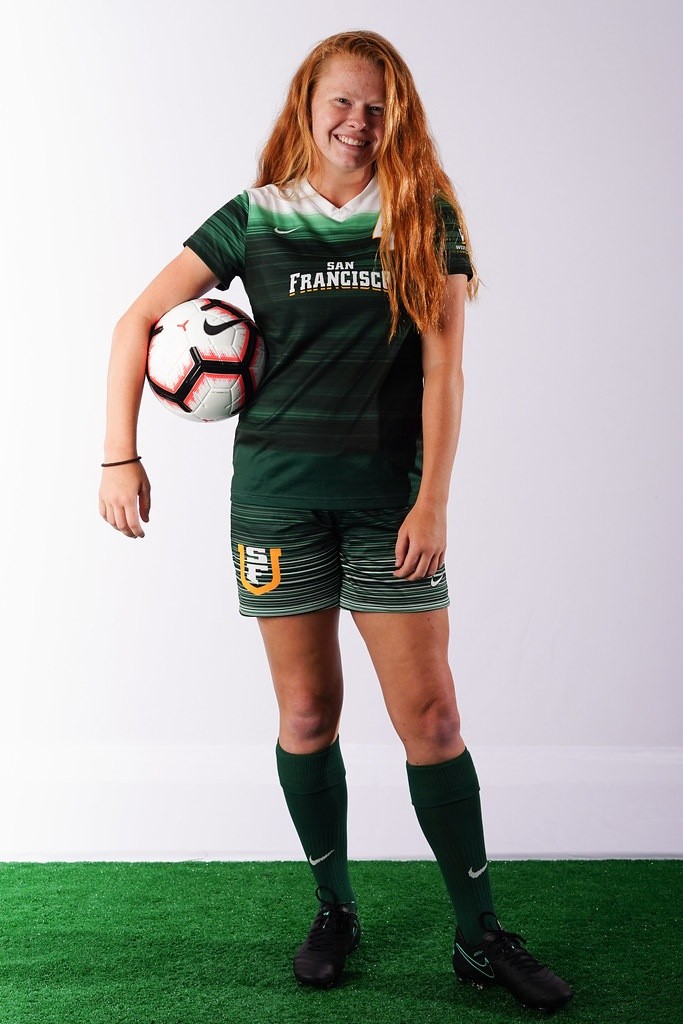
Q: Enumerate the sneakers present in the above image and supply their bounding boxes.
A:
[451,921,574,1011]
[292,892,361,986]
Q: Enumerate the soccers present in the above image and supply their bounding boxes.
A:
[147,298,266,422]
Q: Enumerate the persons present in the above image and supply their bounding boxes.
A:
[98,33,572,1010]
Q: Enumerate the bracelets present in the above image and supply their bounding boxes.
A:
[100,456,142,466]
